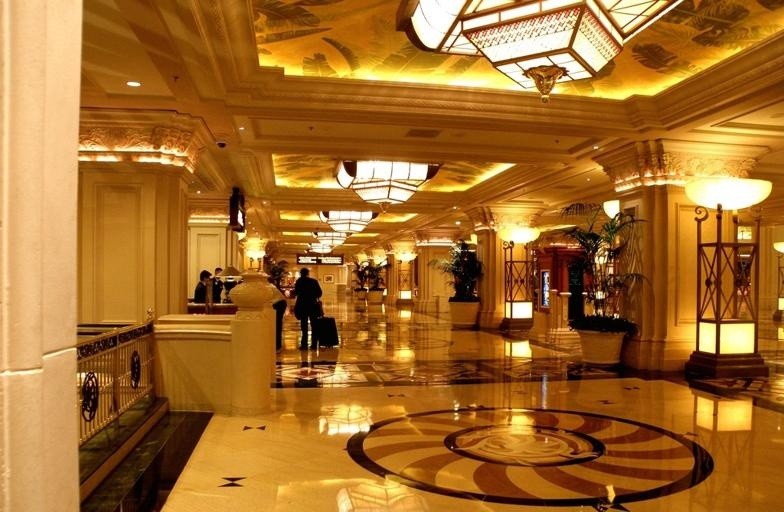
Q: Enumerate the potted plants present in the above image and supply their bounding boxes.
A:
[552,203,651,364]
[351,261,372,299]
[363,258,393,304]
[427,239,487,328]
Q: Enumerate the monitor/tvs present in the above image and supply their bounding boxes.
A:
[230,186,245,233]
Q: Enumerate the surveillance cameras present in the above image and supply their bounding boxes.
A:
[216,142,226,148]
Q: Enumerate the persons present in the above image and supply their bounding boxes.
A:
[211,267,223,303]
[194,270,212,304]
[295,268,322,350]
[266,278,287,353]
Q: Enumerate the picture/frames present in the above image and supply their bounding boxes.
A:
[323,274,335,284]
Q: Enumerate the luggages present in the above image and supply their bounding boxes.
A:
[318,316,339,347]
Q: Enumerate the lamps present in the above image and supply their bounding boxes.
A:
[684,177,772,378]
[395,253,417,307]
[304,160,445,257]
[317,404,373,437]
[773,242,784,322]
[693,390,756,512]
[498,228,541,330]
[503,340,533,436]
[396,0,684,104]
[336,480,429,512]
[215,267,242,304]
[603,200,620,221]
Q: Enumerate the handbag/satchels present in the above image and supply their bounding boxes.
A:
[317,298,324,317]
